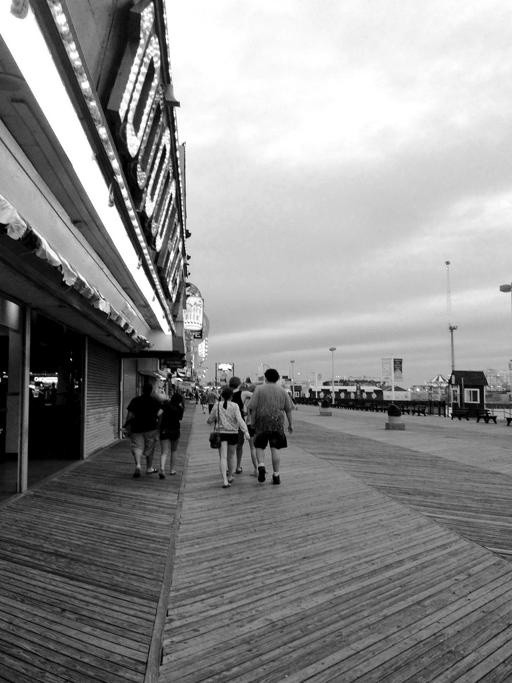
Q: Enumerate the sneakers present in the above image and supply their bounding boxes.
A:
[258,465,266,481]
[273,474,280,484]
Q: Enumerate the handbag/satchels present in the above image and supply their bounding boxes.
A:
[209,432,221,447]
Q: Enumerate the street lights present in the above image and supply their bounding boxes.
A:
[499,281,512,294]
[330,345,338,403]
[448,317,459,372]
[290,359,297,399]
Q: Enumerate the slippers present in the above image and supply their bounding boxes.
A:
[133,467,176,477]
[223,466,242,486]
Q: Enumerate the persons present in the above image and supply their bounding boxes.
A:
[51,383,58,403]
[123,369,296,487]
